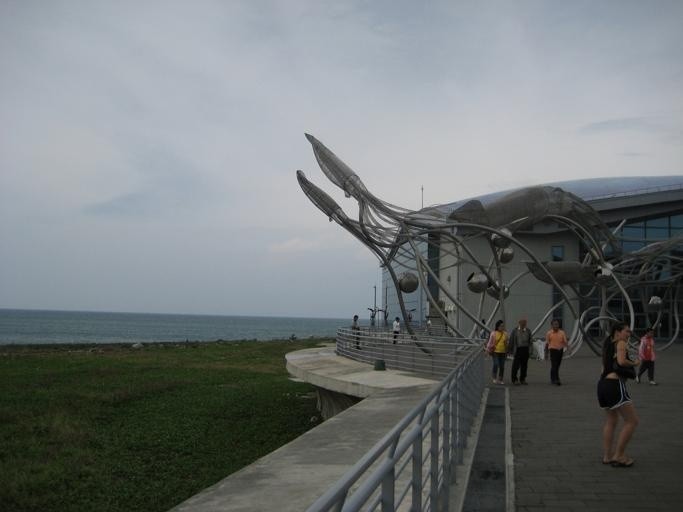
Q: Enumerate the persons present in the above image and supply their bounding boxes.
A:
[426,317,431,335]
[597,320,641,466]
[392,317,401,344]
[635,328,658,385]
[480,318,488,338]
[486,320,508,385]
[508,318,532,385]
[545,319,569,386]
[351,315,362,349]
[624,344,635,385]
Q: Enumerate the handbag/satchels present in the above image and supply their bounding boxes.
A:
[611,341,637,382]
[486,331,496,354]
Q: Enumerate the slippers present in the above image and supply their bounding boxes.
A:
[602,458,634,468]
